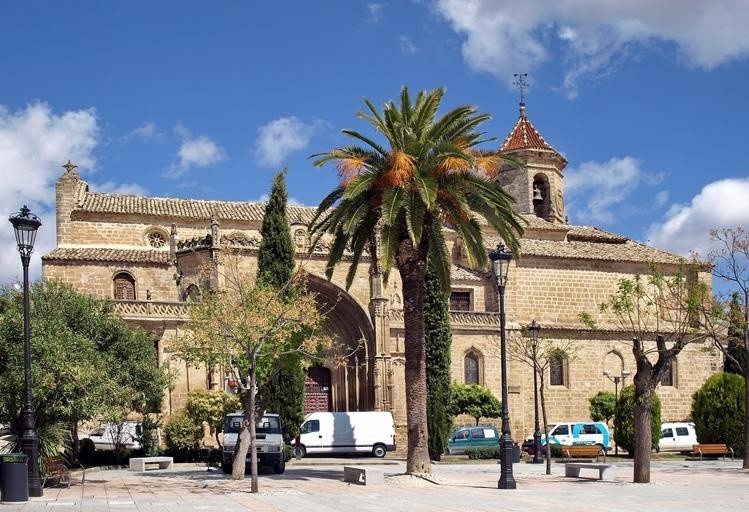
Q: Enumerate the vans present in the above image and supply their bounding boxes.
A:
[521,421,611,456]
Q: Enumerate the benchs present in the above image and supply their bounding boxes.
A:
[129,456,174,472]
[561,445,617,482]
[693,443,735,463]
[344,466,384,487]
[44,455,71,489]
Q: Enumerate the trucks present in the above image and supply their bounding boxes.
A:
[655,419,697,455]
[214,406,289,475]
[75,421,143,458]
[292,409,397,458]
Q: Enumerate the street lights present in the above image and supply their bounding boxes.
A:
[601,369,632,457]
[6,201,49,501]
[527,319,547,465]
[485,240,522,492]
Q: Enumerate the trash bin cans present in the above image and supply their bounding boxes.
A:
[513,442,520,463]
[0,454,29,501]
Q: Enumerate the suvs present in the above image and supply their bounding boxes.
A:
[443,421,501,459]
